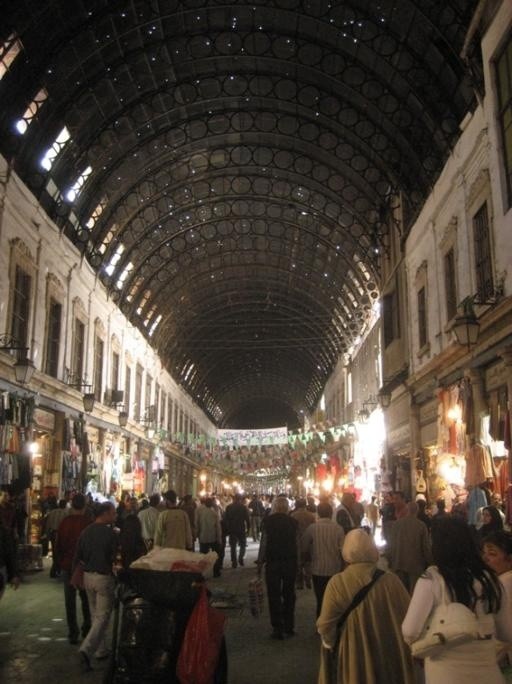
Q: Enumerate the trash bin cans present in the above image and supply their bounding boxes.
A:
[108,568,204,684]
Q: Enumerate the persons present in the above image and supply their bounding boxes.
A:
[0,483,512,683]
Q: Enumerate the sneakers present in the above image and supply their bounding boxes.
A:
[269,629,294,640]
[70,635,111,671]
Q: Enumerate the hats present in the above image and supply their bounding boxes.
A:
[341,528,379,563]
[161,490,176,502]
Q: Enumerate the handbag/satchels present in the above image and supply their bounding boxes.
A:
[410,603,479,661]
